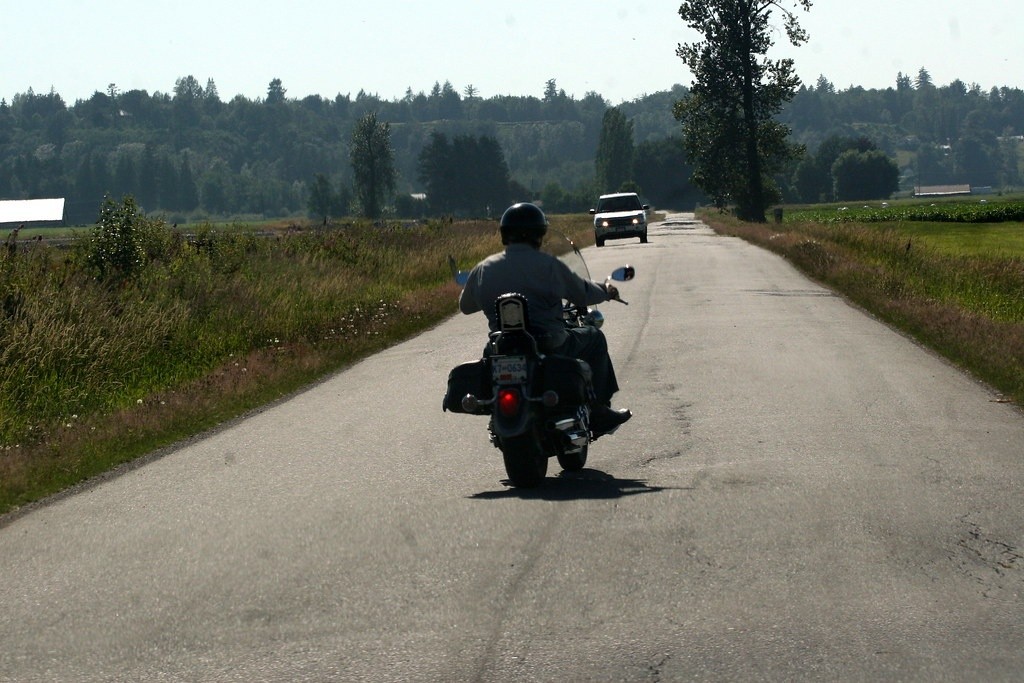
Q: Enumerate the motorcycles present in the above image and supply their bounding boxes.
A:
[454,229,635,488]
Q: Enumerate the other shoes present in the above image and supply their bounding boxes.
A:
[590,406,632,439]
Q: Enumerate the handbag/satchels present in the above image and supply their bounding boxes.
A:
[443,358,492,415]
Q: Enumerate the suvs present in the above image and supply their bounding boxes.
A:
[590,192,651,247]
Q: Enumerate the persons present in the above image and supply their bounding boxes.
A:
[460,202,630,433]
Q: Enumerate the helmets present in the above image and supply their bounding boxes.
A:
[499,203,547,236]
